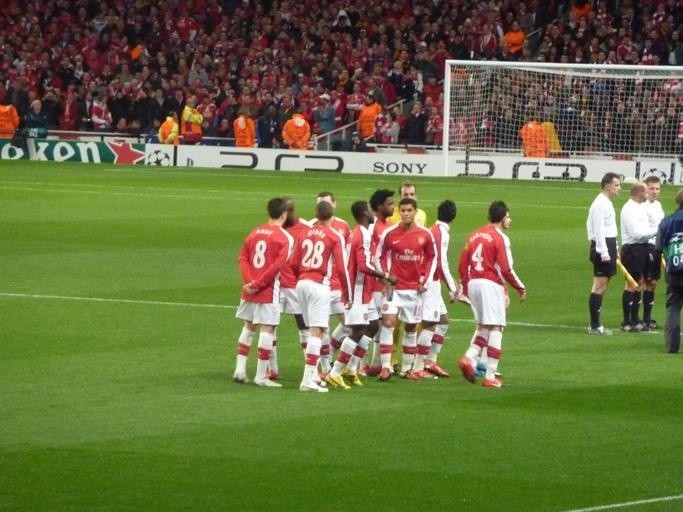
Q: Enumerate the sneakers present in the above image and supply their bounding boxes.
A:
[299,380,328,393]
[341,366,362,386]
[393,365,401,374]
[633,321,646,331]
[620,322,639,332]
[425,362,449,377]
[319,371,330,380]
[376,367,391,381]
[589,325,612,336]
[459,357,476,384]
[325,373,351,390]
[363,365,382,374]
[313,378,327,387]
[254,377,282,387]
[482,377,502,388]
[266,368,278,379]
[477,363,502,376]
[411,369,438,380]
[233,371,250,383]
[647,321,657,331]
[399,371,418,380]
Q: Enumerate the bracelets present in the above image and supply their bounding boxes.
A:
[383,273,389,279]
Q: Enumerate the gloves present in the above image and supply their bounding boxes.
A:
[601,256,610,262]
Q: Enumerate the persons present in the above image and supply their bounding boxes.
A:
[356,94,382,139]
[281,112,311,150]
[233,112,256,147]
[46,116,162,143]
[586,172,621,335]
[386,183,426,367]
[266,196,322,382]
[232,197,294,388]
[655,188,683,353]
[0,1,20,84]
[291,201,353,392]
[298,99,314,141]
[331,90,347,129]
[458,200,526,389]
[641,176,665,328]
[215,0,323,118]
[308,191,351,375]
[346,83,367,124]
[324,201,399,391]
[358,188,396,377]
[3,75,28,118]
[313,93,335,134]
[185,0,214,99]
[373,104,444,149]
[206,118,235,146]
[341,131,368,152]
[196,95,217,131]
[157,110,179,144]
[366,0,445,104]
[14,99,48,138]
[256,105,281,148]
[376,198,438,381]
[20,0,185,117]
[180,97,204,146]
[0,76,12,101]
[0,95,21,139]
[620,181,658,332]
[323,0,366,95]
[410,199,457,379]
[456,208,512,377]
[444,0,683,160]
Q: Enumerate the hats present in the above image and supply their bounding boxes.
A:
[319,93,330,100]
